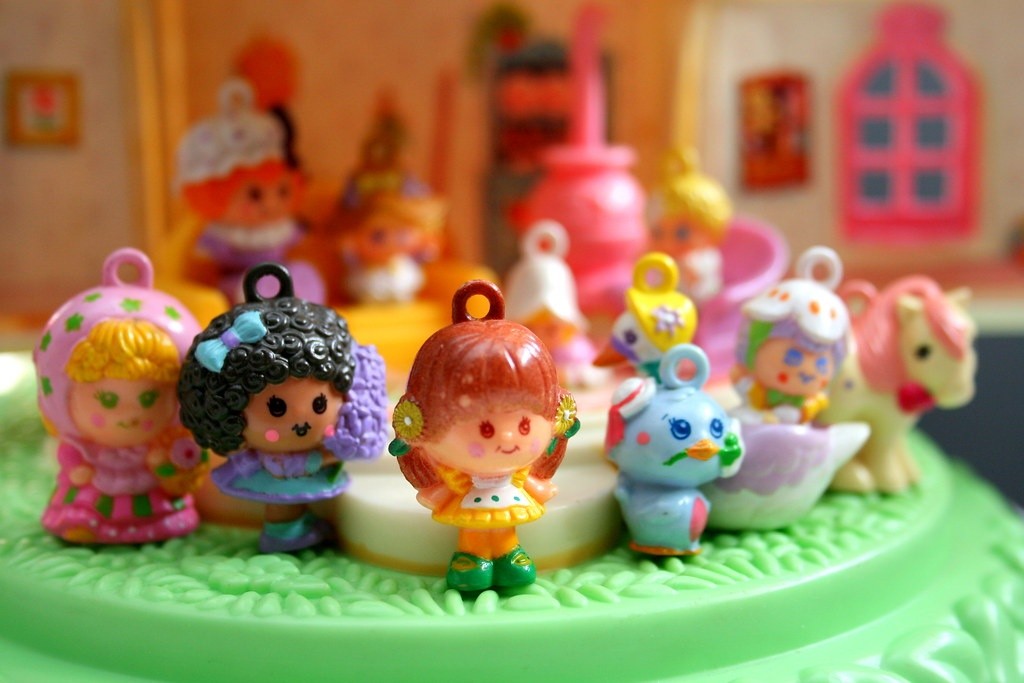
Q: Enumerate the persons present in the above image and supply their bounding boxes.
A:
[175,36,858,592]
[35,249,205,546]
